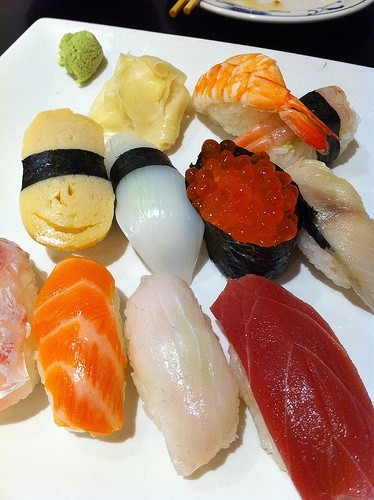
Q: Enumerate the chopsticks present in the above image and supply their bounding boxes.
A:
[169,0,201,18]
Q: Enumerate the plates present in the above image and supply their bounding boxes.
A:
[0,18,374,499]
[192,0,374,24]
